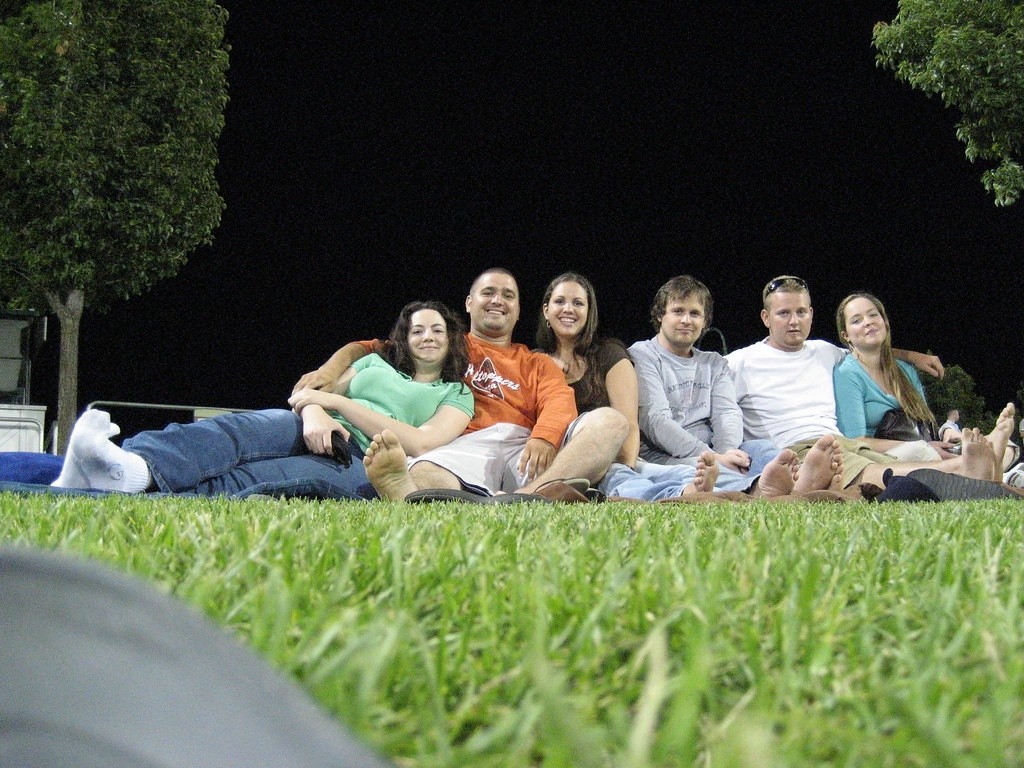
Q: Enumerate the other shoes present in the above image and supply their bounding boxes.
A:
[406,489,545,509]
[563,479,590,495]
[905,469,1020,503]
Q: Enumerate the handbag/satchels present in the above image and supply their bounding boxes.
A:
[874,408,931,441]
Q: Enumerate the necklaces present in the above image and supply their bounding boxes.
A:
[556,351,574,374]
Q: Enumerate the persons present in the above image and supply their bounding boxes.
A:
[50,300,474,496]
[531,272,1024,501]
[291,267,630,501]
[0,546,391,768]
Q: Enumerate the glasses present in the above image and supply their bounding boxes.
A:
[765,278,808,300]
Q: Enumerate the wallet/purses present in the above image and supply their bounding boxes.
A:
[316,431,353,468]
